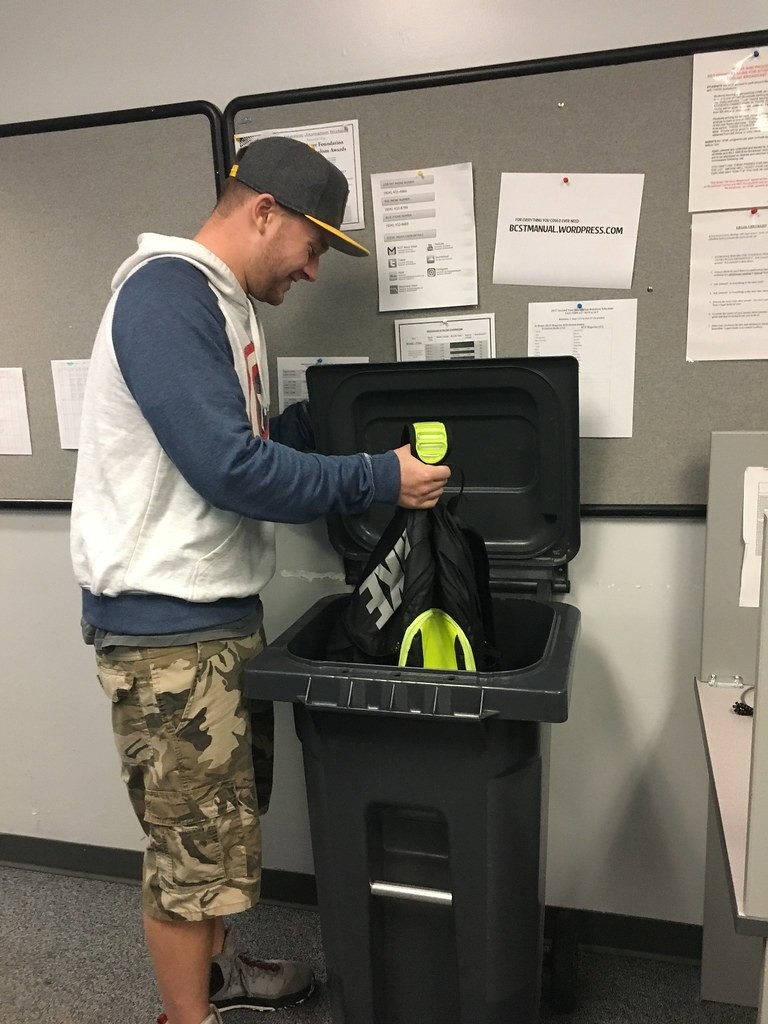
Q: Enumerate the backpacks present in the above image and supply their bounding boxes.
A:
[321,421,504,673]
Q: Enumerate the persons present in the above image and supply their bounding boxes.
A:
[69,135,452,1023]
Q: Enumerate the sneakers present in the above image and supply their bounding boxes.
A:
[156,922,316,1023]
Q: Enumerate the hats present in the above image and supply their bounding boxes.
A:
[229,136,370,258]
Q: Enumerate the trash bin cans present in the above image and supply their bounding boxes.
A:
[241,354,582,1024]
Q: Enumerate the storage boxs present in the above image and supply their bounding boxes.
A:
[240,352,582,1022]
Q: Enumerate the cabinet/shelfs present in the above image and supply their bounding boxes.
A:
[692,428,768,1024]
